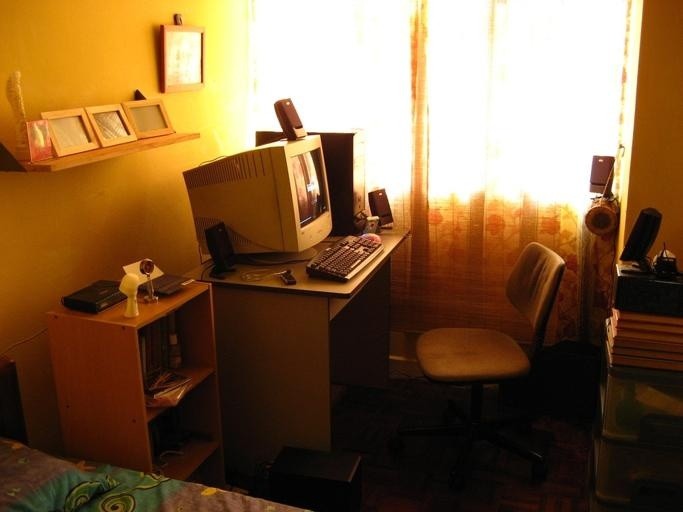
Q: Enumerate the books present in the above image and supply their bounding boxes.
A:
[604,307,683,371]
[140,307,191,407]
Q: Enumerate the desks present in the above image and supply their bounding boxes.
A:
[181,224,413,457]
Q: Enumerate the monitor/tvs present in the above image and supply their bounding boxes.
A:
[183,134,333,264]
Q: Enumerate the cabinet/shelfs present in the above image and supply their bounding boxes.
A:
[43,278,229,489]
[591,342,681,512]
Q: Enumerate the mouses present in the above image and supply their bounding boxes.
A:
[361,233,381,244]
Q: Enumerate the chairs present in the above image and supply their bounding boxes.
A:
[409,239,566,469]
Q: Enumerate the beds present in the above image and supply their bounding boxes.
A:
[0,353,314,511]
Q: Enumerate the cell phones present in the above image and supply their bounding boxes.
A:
[281,274,296,284]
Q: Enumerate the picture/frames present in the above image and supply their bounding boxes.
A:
[120,98,176,140]
[39,108,99,158]
[159,23,205,94]
[85,104,137,150]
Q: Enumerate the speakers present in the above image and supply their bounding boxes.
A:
[274,98,306,139]
[590,155,615,202]
[204,223,235,279]
[620,207,662,274]
[368,188,394,229]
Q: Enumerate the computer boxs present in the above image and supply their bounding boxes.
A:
[256,131,368,235]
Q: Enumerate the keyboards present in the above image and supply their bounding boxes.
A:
[306,236,383,282]
